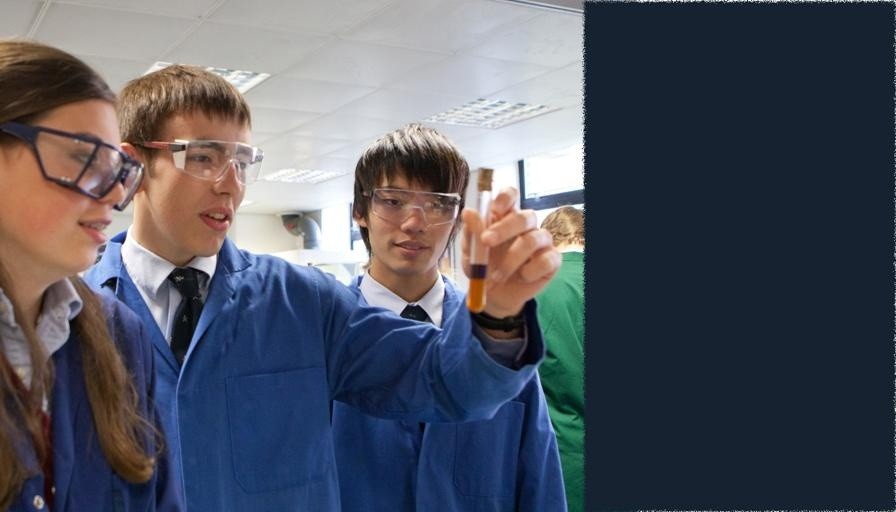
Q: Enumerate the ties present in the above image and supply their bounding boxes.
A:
[401,306,427,322]
[169,267,205,368]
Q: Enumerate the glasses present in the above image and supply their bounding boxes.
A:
[132,137,264,187]
[362,188,462,227]
[1,120,145,212]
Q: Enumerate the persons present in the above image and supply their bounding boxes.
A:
[80,64,563,510]
[329,122,568,511]
[532,207,586,511]
[1,41,156,512]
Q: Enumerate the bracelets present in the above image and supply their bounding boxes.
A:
[473,311,524,333]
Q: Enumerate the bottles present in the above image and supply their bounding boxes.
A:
[467,169,493,314]
